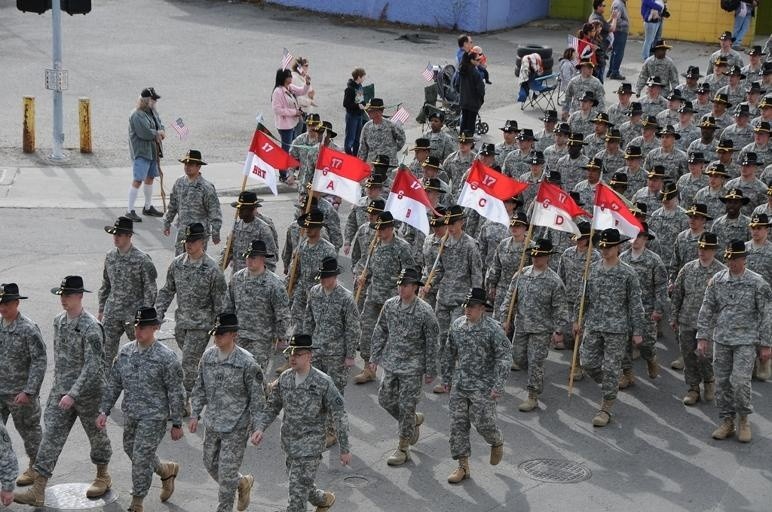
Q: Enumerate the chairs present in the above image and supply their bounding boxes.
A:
[521,53,561,113]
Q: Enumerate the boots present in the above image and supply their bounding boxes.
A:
[236,474,254,511]
[314,491,335,511]
[387,437,412,467]
[447,455,471,484]
[276,362,291,374]
[517,352,660,427]
[86,464,112,499]
[325,434,337,448]
[159,462,179,502]
[669,354,771,443]
[490,442,504,467]
[353,366,377,383]
[13,466,48,507]
[127,496,143,511]
[433,384,444,394]
[409,413,425,445]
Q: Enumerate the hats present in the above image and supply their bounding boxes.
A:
[105,217,135,236]
[140,87,161,99]
[178,150,207,167]
[51,275,92,296]
[240,240,275,259]
[181,223,207,244]
[208,313,240,336]
[0,284,29,303]
[231,191,263,209]
[283,334,313,355]
[125,306,161,327]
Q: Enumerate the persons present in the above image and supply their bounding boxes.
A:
[588,0,621,83]
[591,20,614,84]
[607,0,629,80]
[370,30,772,486]
[1,275,351,511]
[642,0,667,63]
[578,23,597,70]
[94,49,415,449]
[730,0,759,51]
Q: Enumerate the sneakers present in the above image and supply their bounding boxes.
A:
[126,210,142,223]
[143,205,164,218]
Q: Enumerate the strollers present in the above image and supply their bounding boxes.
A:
[434,63,489,137]
